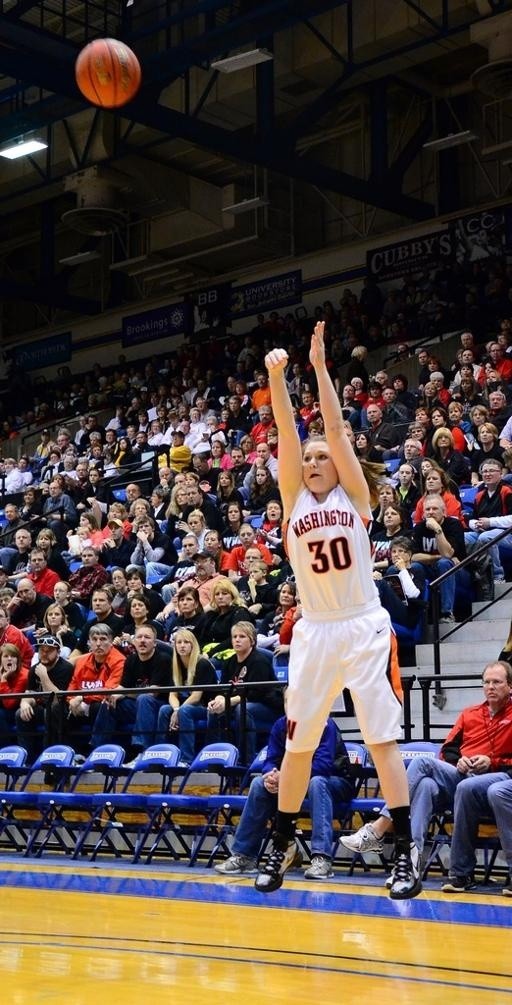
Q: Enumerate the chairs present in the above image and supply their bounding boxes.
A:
[392,577,429,666]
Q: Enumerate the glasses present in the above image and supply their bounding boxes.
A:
[480,468,501,473]
[36,637,59,646]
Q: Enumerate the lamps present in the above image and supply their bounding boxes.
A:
[221,158,270,214]
[422,45,479,152]
[211,0,274,73]
[58,215,103,266]
[0,130,49,159]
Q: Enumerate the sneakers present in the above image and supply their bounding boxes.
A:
[442,873,478,891]
[493,577,506,584]
[503,877,512,895]
[304,855,335,879]
[256,841,303,892]
[390,840,425,897]
[438,611,457,624]
[214,853,257,874]
[339,822,386,855]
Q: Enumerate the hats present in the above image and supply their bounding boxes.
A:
[171,429,184,437]
[33,634,60,648]
[107,518,122,526]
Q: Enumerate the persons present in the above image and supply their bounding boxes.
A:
[1,259,512,900]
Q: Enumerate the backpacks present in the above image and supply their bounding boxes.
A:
[464,541,495,601]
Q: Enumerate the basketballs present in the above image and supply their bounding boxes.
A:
[76,38,142,108]
